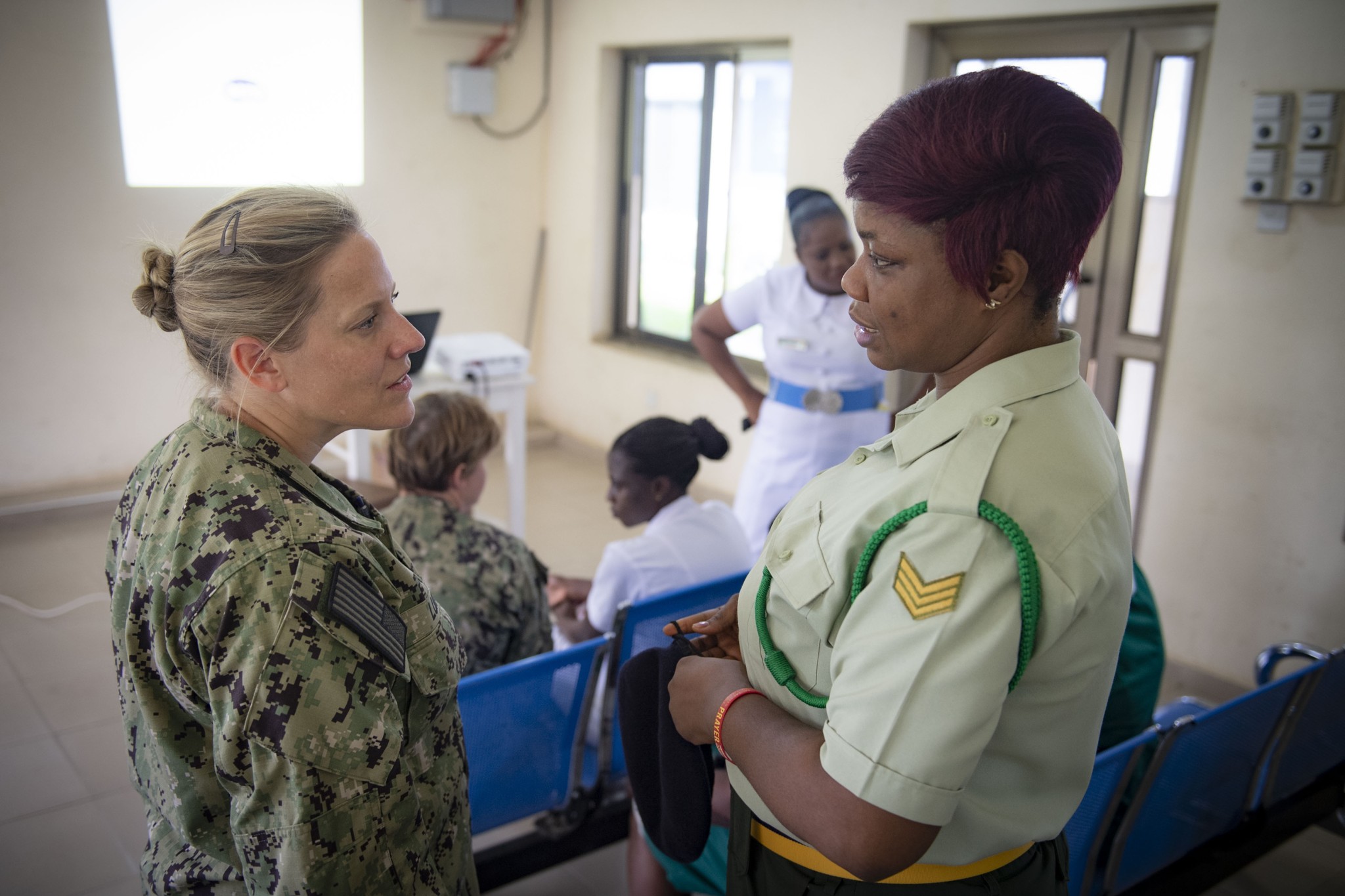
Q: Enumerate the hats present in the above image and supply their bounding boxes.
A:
[615,646,715,863]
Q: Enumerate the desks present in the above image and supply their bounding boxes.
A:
[315,332,530,541]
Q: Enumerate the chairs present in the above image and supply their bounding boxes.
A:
[455,570,750,896]
[1064,640,1345,896]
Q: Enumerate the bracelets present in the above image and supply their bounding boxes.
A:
[713,687,769,766]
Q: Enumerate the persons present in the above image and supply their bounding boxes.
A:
[105,187,484,896]
[546,414,753,746]
[668,65,1136,896]
[688,186,921,565]
[371,390,554,682]
